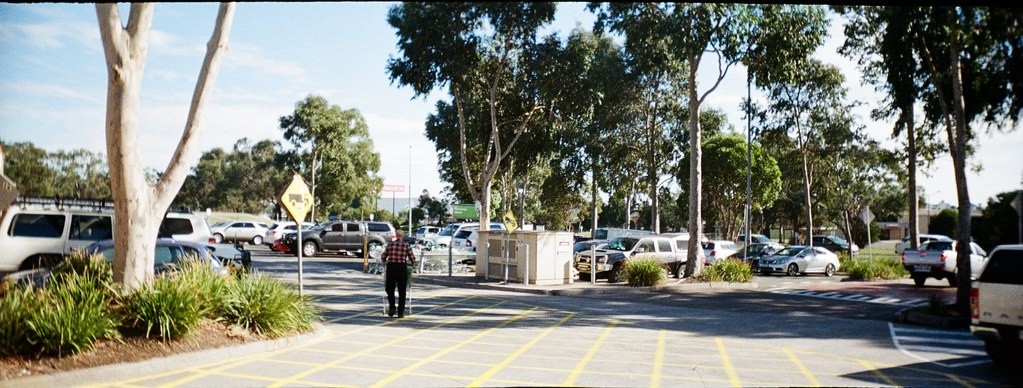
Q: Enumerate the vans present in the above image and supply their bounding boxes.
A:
[574,233,708,279]
[436,223,506,249]
[416,226,444,240]
[702,241,739,264]
[283,220,401,258]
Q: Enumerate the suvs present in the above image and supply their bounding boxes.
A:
[0,195,245,274]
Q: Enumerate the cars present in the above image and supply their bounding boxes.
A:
[738,234,772,245]
[895,234,951,256]
[757,245,840,277]
[452,228,477,251]
[902,239,990,285]
[805,236,860,256]
[167,212,217,244]
[263,223,314,252]
[10,239,232,289]
[969,245,1023,371]
[209,220,269,246]
[729,243,789,270]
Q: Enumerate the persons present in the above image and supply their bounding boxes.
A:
[382,230,416,318]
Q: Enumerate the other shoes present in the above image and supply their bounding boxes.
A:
[388,310,396,317]
[398,313,404,317]
